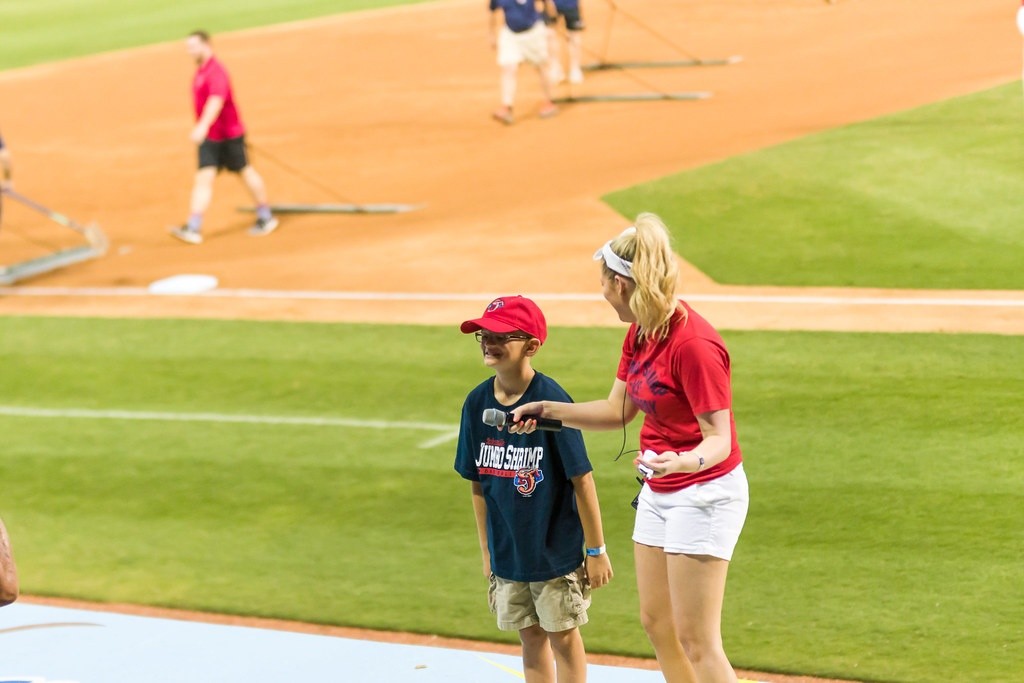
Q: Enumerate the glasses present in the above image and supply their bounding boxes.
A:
[475,332,531,345]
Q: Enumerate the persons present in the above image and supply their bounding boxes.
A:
[168,29,278,242]
[454,295,614,683]
[495,213,749,683]
[486,0,585,123]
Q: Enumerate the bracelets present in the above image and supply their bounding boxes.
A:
[586,544,605,556]
[680,451,704,472]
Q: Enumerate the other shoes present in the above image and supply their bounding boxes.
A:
[249,218,279,236]
[568,69,583,83]
[171,225,202,244]
[542,107,559,118]
[493,112,512,124]
[551,66,563,82]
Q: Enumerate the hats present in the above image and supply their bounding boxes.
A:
[460,294,546,346]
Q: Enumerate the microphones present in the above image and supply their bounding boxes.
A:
[481,408,563,432]
